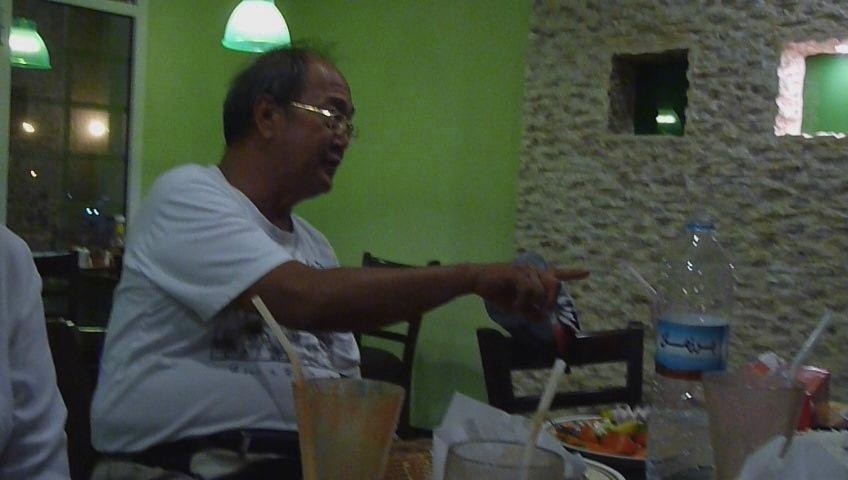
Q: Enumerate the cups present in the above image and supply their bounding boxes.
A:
[442,439,566,480]
[701,370,808,480]
[291,377,405,480]
[90,247,106,268]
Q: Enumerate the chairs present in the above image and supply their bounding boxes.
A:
[477,322,643,412]
[47,318,108,480]
[356,253,441,439]
[45,262,118,329]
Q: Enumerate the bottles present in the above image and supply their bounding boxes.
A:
[642,211,734,480]
[110,214,127,269]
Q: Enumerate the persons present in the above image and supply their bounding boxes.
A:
[90,47,589,480]
[0,223,70,478]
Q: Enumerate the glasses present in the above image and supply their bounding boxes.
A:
[286,100,359,143]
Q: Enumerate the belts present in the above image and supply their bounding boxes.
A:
[159,429,301,459]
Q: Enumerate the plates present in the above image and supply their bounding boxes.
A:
[576,457,626,480]
[543,413,676,463]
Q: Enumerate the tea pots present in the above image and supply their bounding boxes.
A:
[77,247,93,269]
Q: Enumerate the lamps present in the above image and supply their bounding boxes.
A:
[8,18,51,70]
[221,0,292,53]
